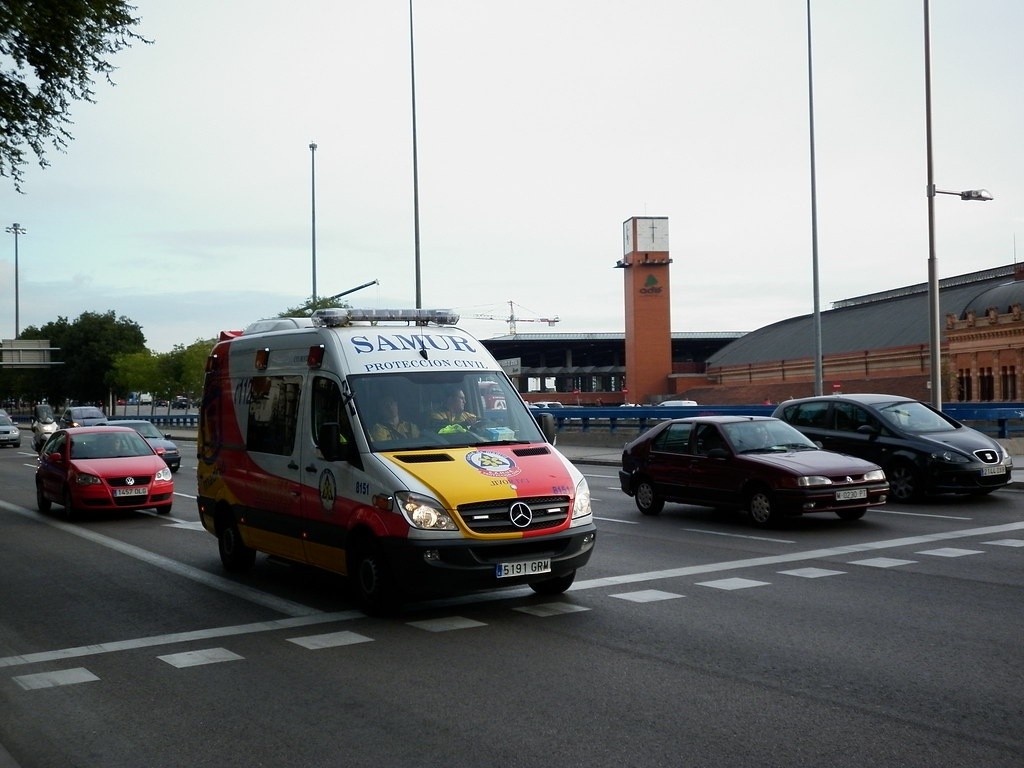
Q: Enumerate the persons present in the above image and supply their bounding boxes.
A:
[759,397,772,405]
[32,409,60,433]
[595,397,604,407]
[107,437,121,457]
[431,386,481,434]
[370,390,420,442]
[751,424,792,449]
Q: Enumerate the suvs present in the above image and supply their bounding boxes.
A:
[768,393,1012,506]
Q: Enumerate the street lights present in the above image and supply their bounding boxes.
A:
[6,222,26,340]
[929,189,995,414]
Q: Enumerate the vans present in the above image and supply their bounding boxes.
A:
[194,308,598,618]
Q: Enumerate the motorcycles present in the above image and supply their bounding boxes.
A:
[31,405,61,454]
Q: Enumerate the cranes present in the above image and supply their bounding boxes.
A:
[460,301,561,335]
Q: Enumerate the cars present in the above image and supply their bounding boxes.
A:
[118,391,190,410]
[106,420,182,473]
[35,426,174,518]
[60,406,108,428]
[0,408,21,448]
[526,400,698,423]
[617,414,891,530]
